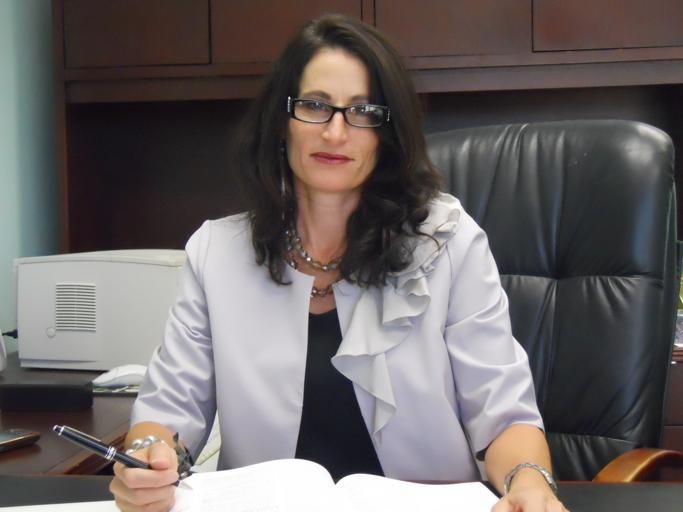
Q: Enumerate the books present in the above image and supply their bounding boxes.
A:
[179,459,501,509]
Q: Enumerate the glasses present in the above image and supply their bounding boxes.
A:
[288,96,390,127]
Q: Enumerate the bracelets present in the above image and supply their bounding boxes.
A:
[124,432,163,451]
[501,462,560,497]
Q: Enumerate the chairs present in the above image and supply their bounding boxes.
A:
[420,118,682,481]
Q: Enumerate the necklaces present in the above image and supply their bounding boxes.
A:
[284,232,346,300]
[281,218,351,273]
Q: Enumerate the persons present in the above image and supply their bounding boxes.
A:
[103,12,567,510]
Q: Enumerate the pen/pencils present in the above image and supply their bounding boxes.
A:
[53,424,195,493]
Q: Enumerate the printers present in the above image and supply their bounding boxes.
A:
[14,250,186,370]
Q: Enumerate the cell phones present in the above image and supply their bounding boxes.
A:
[0,429,41,452]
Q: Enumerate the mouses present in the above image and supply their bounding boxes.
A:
[91,363,150,388]
